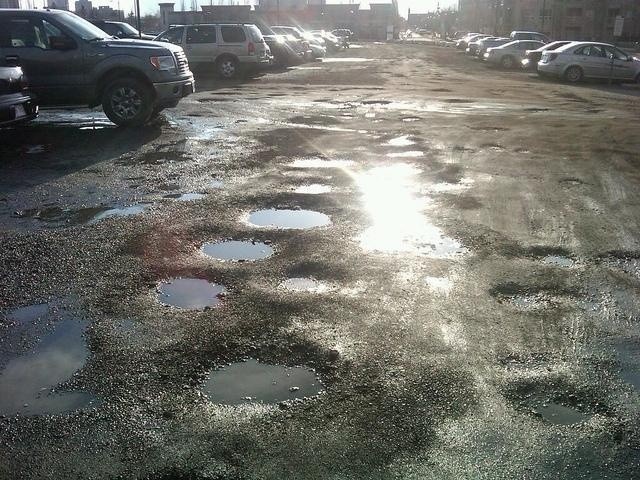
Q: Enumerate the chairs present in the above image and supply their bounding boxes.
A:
[11,21,39,45]
[601,47,607,58]
[531,43,535,49]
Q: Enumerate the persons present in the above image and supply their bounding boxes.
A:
[343,29,347,38]
[343,37,349,48]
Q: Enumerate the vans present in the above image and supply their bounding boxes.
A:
[151,22,276,79]
[419,28,432,36]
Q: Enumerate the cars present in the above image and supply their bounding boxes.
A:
[0,55,39,132]
[537,41,640,82]
[452,30,551,69]
[520,41,571,71]
[263,23,354,72]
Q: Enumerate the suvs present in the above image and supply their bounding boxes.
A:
[1,7,197,127]
[86,19,168,41]
